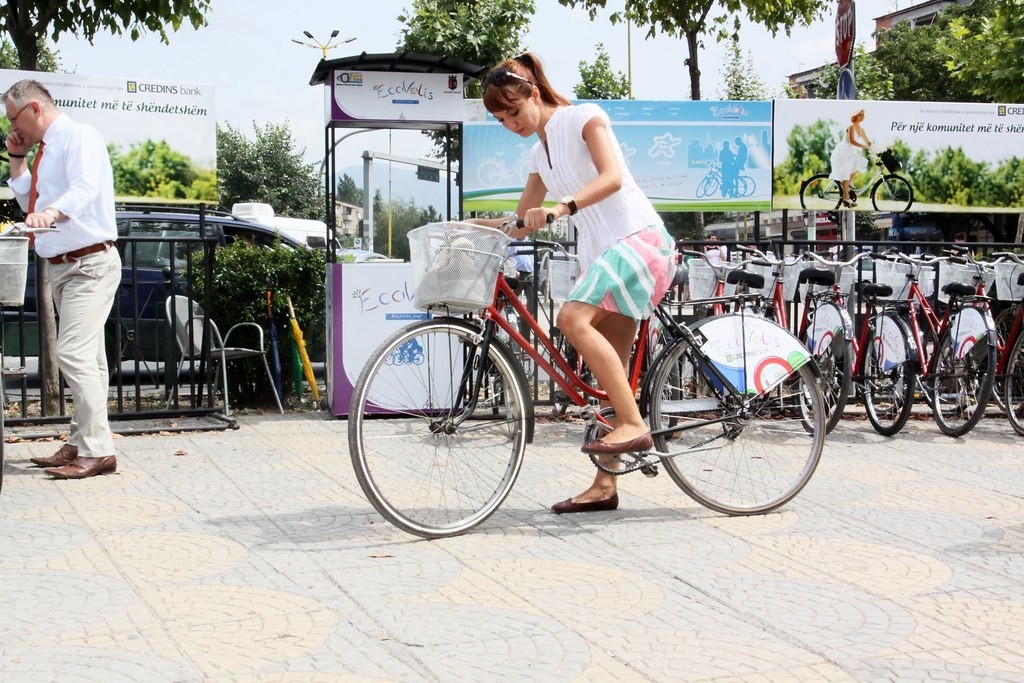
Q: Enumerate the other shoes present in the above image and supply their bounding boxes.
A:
[551,492,619,514]
[843,198,858,207]
[581,430,654,455]
[849,183,856,190]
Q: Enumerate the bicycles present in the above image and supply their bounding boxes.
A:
[678,244,1024,441]
[346,212,828,541]
[798,144,914,211]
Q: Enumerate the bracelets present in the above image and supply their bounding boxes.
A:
[8,152,25,158]
[43,209,57,220]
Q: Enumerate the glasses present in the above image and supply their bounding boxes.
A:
[8,101,41,123]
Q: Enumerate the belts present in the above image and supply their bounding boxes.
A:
[47,240,114,265]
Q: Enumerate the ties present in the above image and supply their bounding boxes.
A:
[27,140,46,248]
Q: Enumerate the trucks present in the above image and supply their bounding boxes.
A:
[224,202,344,250]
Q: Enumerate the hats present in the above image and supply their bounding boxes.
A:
[850,105,863,116]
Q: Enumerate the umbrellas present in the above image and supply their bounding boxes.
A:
[287,296,321,407]
[266,290,283,408]
[290,314,303,404]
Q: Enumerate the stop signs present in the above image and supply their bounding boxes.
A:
[835,0,855,69]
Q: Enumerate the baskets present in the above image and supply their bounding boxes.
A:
[746,256,800,301]
[874,258,934,300]
[799,261,856,307]
[548,260,580,301]
[994,262,1024,301]
[938,260,996,307]
[687,258,744,299]
[881,148,902,174]
[406,221,511,316]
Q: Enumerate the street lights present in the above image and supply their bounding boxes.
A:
[291,29,358,60]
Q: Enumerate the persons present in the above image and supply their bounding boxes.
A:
[505,235,534,318]
[670,235,732,308]
[1,80,123,480]
[830,106,872,207]
[462,53,676,512]
[950,233,974,259]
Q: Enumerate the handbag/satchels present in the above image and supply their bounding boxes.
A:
[414,235,486,313]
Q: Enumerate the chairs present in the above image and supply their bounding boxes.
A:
[165,294,285,418]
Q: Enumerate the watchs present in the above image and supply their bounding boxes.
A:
[561,196,578,215]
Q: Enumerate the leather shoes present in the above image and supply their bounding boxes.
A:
[31,443,117,479]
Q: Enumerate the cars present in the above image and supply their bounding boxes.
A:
[1,206,392,361]
[334,250,387,263]
